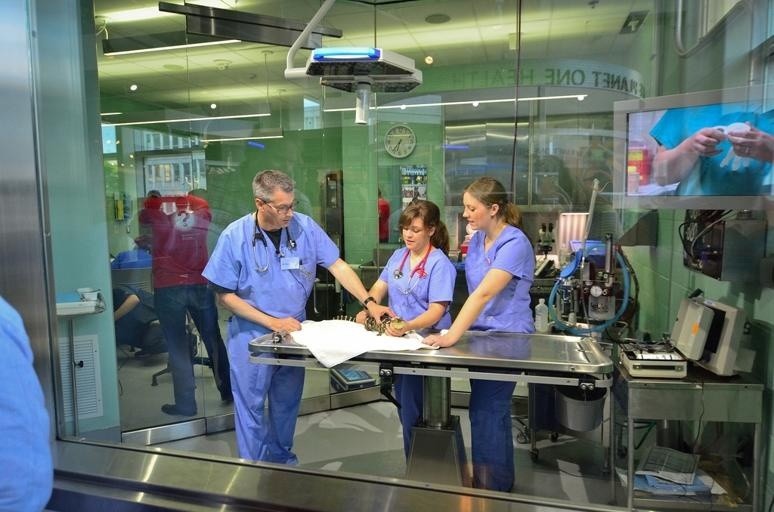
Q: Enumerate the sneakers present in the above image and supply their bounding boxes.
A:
[188,332,199,357]
[135,350,152,357]
[161,404,180,415]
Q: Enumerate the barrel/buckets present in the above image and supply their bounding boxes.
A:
[554,390,607,432]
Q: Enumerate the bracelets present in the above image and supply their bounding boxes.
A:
[361,298,375,309]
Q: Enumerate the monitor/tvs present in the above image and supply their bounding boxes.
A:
[691,297,746,377]
[613,80,774,208]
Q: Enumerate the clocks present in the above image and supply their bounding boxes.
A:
[382,124,417,160]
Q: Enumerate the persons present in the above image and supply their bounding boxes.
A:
[0,294,55,512]
[200,167,396,468]
[140,176,234,416]
[353,199,458,471]
[647,103,774,195]
[113,283,197,358]
[420,176,537,494]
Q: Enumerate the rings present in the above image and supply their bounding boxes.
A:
[745,146,750,157]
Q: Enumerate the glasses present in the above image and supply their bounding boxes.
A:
[262,200,299,215]
